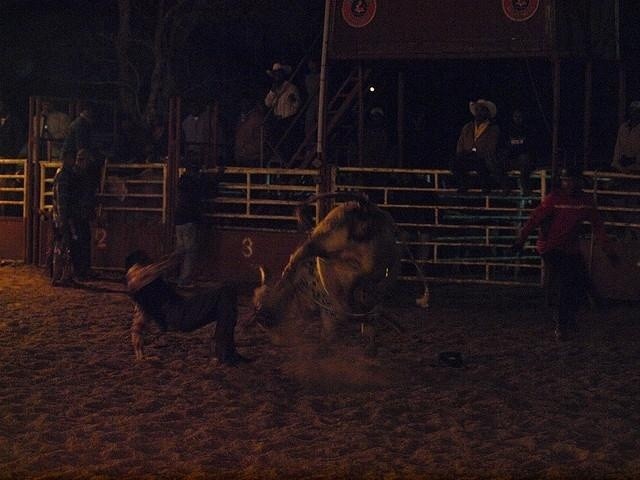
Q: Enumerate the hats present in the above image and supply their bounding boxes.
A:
[468,99,497,119]
[432,351,464,371]
[265,62,293,77]
[548,166,589,189]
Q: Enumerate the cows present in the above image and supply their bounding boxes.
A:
[255,191,395,359]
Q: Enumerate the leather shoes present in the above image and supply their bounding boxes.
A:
[214,352,258,371]
[553,320,568,342]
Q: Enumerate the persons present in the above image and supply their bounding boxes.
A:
[1,58,320,287]
[515,161,614,345]
[452,98,543,198]
[113,245,275,368]
[612,99,640,193]
